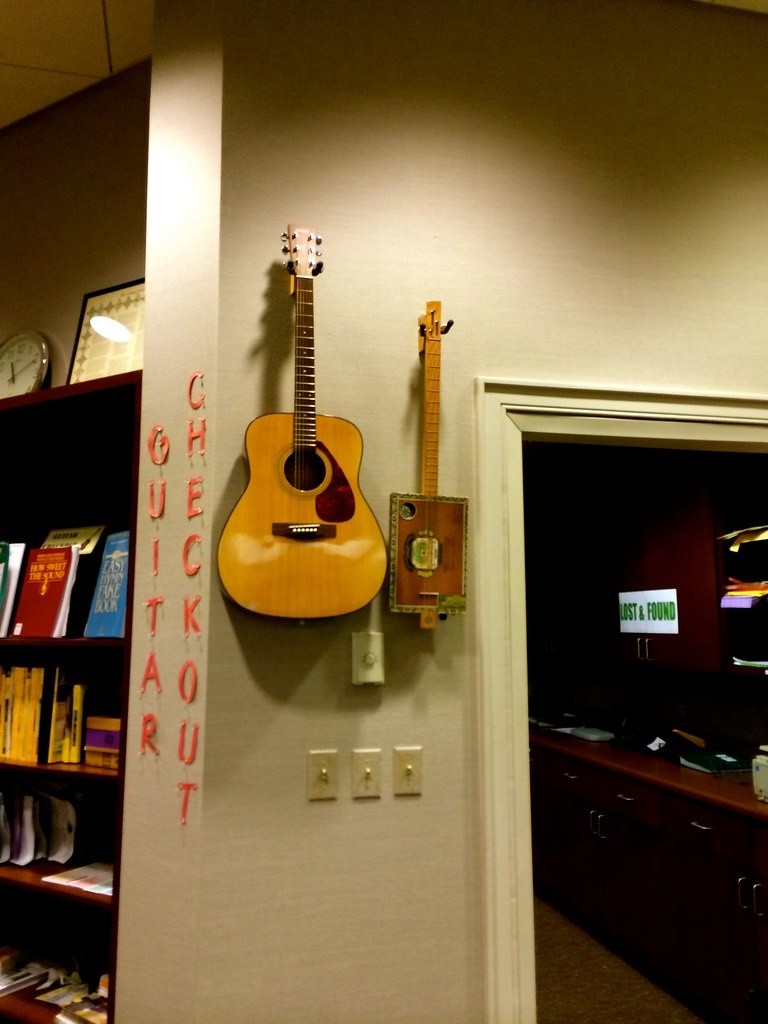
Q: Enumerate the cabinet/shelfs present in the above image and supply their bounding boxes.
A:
[625,470,768,677]
[529,741,768,1024]
[0,370,142,1024]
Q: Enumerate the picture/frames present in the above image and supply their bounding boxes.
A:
[67,277,146,385]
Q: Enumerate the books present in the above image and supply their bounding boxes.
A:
[0,530,130,772]
[680,752,755,774]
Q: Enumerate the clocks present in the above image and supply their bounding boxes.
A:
[0,331,48,400]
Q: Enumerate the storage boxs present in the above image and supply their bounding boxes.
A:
[83,716,121,768]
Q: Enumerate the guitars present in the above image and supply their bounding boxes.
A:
[216,223,388,618]
[389,301,468,629]
